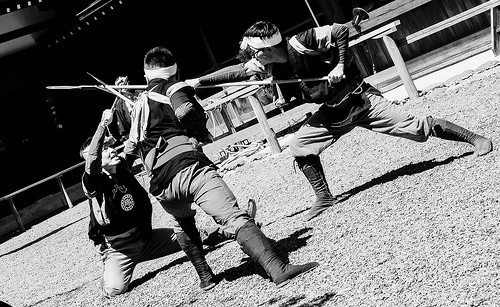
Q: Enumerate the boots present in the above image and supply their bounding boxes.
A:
[233,219,318,287]
[426,115,493,155]
[175,230,219,289]
[293,153,338,221]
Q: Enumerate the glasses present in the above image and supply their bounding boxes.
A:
[249,49,262,59]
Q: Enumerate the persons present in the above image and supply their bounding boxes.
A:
[116,45,319,290]
[81,76,256,297]
[184,22,492,222]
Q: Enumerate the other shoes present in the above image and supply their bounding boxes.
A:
[244,198,257,220]
